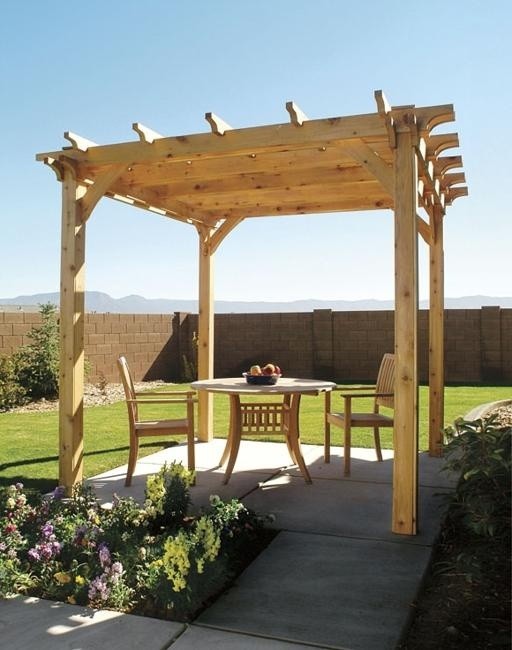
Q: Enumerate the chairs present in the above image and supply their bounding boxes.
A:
[323,352,397,477]
[116,355,199,487]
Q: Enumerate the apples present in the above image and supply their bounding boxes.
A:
[251,363,280,376]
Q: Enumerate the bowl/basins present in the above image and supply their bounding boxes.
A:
[241,372,282,385]
